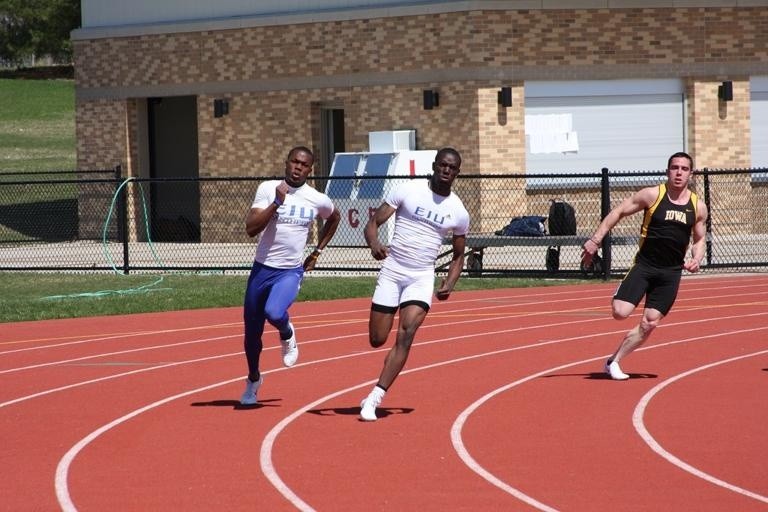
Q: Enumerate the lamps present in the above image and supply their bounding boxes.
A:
[497,85,513,108]
[718,80,734,103]
[422,88,439,110]
[214,98,229,118]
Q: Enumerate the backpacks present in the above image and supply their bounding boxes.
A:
[548,202,576,235]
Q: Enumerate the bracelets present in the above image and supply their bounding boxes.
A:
[588,235,602,249]
[310,252,319,260]
[272,198,282,207]
[315,247,325,255]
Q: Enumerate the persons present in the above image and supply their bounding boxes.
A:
[241,145,343,408]
[578,152,710,382]
[359,145,472,422]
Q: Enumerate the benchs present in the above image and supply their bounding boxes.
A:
[442,231,628,280]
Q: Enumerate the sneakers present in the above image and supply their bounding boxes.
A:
[604,358,630,380]
[360,397,378,421]
[240,375,263,405]
[280,323,299,367]
